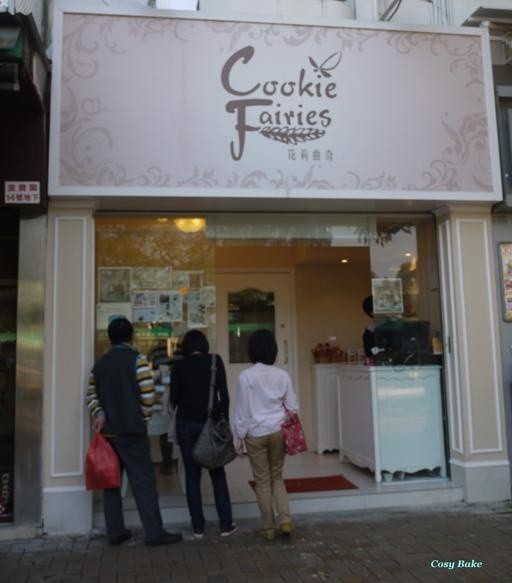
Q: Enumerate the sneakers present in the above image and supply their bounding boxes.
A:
[192,530,205,538]
[220,520,238,536]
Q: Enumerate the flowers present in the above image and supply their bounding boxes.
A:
[311,342,345,363]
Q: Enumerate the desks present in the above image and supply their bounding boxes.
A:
[309,363,336,458]
[337,359,448,486]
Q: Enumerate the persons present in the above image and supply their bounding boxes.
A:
[230,328,300,539]
[358,291,382,358]
[84,316,182,548]
[168,326,238,539]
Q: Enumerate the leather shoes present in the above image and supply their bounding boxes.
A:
[108,528,132,545]
[257,527,275,540]
[279,523,292,537]
[145,528,182,546]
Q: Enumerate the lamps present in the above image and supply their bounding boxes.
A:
[172,217,206,233]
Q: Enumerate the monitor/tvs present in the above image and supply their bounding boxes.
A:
[375,320,429,352]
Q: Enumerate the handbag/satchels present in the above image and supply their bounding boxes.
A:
[280,402,308,456]
[190,418,237,471]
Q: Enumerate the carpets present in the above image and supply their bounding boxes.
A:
[248,474,358,492]
